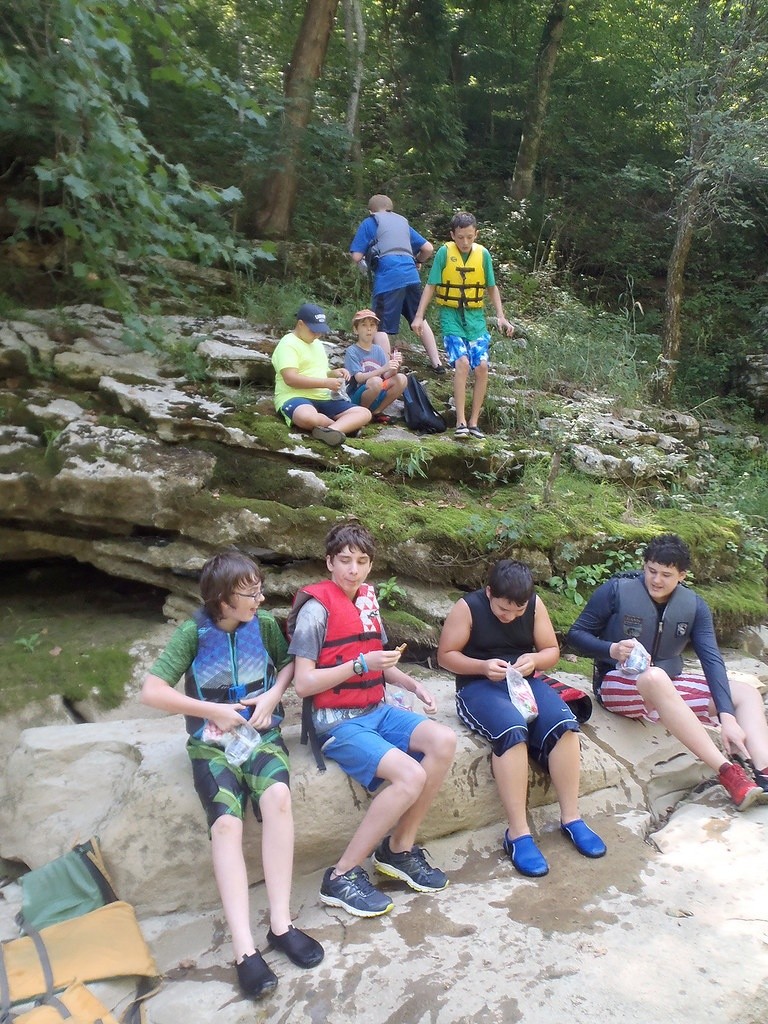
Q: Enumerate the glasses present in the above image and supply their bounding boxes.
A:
[231,588,264,601]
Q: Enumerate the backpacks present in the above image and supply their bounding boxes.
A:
[404,374,447,435]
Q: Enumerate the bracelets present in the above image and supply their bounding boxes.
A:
[360,653,368,673]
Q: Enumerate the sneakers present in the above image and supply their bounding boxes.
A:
[371,836,449,892]
[760,767,768,781]
[319,865,394,918]
[717,762,764,811]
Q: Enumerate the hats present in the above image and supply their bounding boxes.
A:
[352,310,380,323]
[297,304,330,333]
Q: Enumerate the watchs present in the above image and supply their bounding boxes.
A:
[353,657,364,677]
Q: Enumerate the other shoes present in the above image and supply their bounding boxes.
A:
[468,426,485,439]
[266,924,324,969]
[427,363,446,373]
[503,827,548,877]
[346,430,362,438]
[311,426,346,446]
[235,948,278,1000]
[454,423,470,438]
[374,413,395,425]
[560,819,606,858]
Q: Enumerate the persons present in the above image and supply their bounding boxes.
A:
[569,533,768,811]
[272,303,373,446]
[286,519,456,917]
[139,552,325,996]
[411,212,514,440]
[438,559,608,878]
[345,309,408,421]
[350,194,446,374]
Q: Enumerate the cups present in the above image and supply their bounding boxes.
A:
[226,723,261,766]
